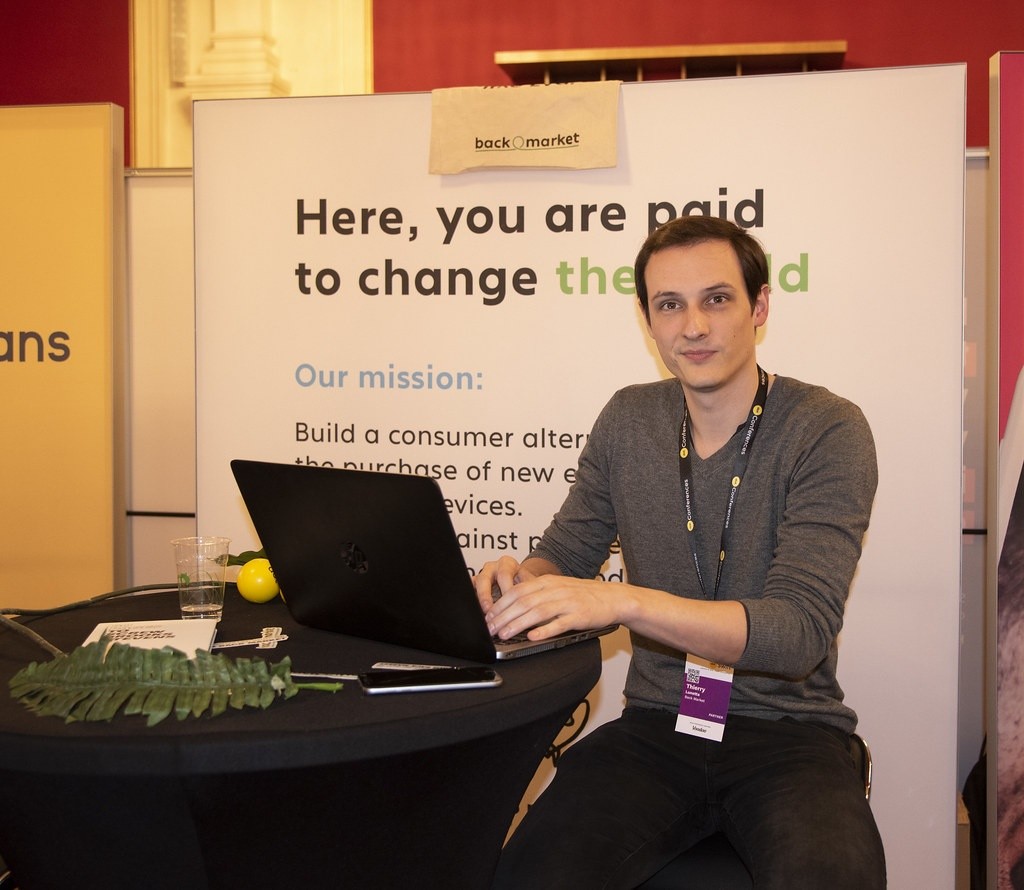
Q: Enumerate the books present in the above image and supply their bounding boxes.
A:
[80,619,218,661]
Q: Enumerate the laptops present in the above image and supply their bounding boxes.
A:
[231,459,620,664]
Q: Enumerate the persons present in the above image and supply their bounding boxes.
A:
[472,215,887,887]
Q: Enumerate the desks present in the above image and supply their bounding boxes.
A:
[1,587,601,890]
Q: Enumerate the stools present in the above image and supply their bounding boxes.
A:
[635,735,873,890]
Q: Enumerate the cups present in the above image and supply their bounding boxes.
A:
[170,537,233,623]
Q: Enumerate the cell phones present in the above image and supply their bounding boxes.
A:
[358,665,502,695]
